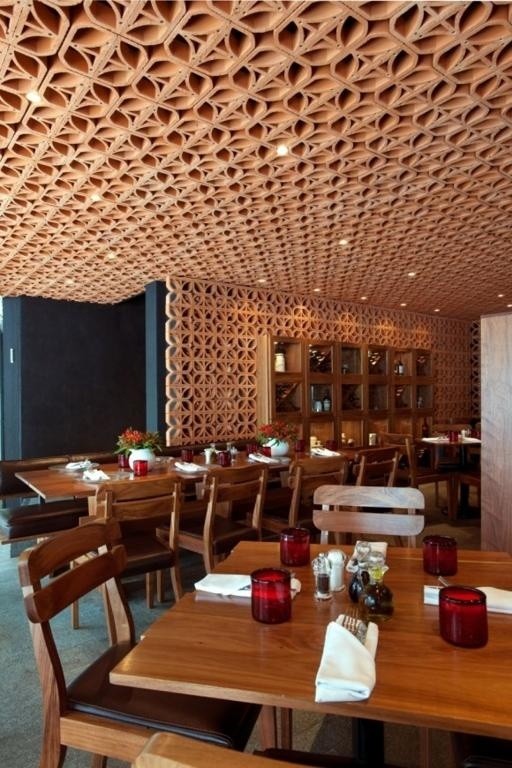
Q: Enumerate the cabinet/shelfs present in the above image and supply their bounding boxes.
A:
[255,333,437,449]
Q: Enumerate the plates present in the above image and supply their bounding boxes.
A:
[47,462,132,485]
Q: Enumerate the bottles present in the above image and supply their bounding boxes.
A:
[311,388,332,412]
[274,353,287,373]
[421,418,430,437]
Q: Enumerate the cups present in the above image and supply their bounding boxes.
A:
[116,438,339,476]
[394,364,405,375]
[449,431,459,441]
[248,526,489,648]
